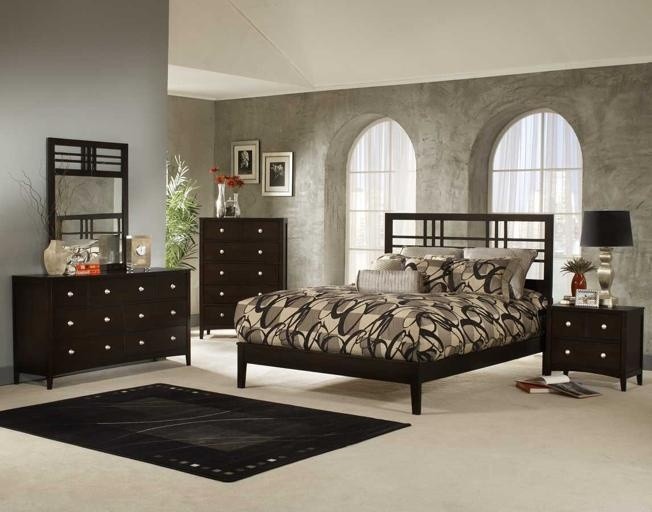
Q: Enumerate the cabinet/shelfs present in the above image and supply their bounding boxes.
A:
[542,302,646,392]
[12,267,192,390]
[199,217,288,339]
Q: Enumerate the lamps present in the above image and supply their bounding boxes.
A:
[579,210,635,308]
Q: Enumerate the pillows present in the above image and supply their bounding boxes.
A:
[357,246,538,303]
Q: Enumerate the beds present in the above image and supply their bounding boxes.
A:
[234,213,554,415]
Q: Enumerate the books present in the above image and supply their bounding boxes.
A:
[513,375,600,398]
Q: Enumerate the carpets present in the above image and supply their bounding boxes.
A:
[1,383,410,483]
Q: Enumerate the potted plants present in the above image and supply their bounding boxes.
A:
[559,255,598,298]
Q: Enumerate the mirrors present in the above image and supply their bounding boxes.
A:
[46,137,129,271]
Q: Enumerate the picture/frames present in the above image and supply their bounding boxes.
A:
[261,152,295,197]
[575,289,599,307]
[230,140,260,184]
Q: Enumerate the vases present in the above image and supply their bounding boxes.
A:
[231,186,242,218]
[214,184,226,218]
[209,164,229,186]
[226,173,245,190]
[42,239,67,275]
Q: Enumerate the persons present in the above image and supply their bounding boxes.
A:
[583,294,588,304]
